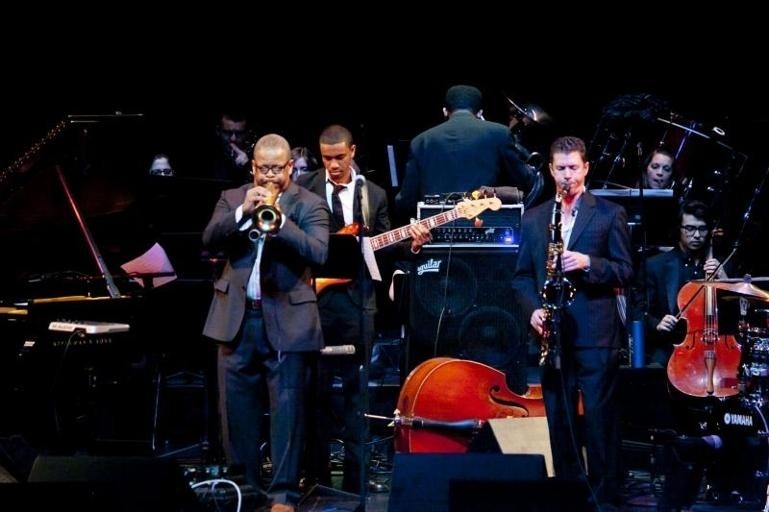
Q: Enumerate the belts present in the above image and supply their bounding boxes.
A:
[246,298,262,311]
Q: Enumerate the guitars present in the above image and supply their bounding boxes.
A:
[309,192,502,298]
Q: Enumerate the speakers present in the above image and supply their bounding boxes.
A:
[406,250,529,396]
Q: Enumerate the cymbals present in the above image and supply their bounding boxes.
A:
[690,280,769,300]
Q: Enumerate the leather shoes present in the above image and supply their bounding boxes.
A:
[369,480,388,492]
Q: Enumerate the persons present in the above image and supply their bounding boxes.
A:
[147,153,177,179]
[633,197,728,498]
[201,132,331,512]
[210,110,257,185]
[624,145,686,336]
[395,83,543,397]
[295,124,434,511]
[511,134,634,510]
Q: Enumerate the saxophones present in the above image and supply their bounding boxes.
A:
[539,184,576,368]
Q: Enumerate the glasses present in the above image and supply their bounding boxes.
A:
[681,225,709,236]
[254,158,291,174]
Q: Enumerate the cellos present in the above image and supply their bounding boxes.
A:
[395,356,678,457]
[667,219,755,398]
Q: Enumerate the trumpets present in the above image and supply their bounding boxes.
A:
[251,182,283,237]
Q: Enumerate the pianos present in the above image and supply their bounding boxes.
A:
[0,110,219,479]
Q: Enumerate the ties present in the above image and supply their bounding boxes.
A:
[327,179,346,229]
